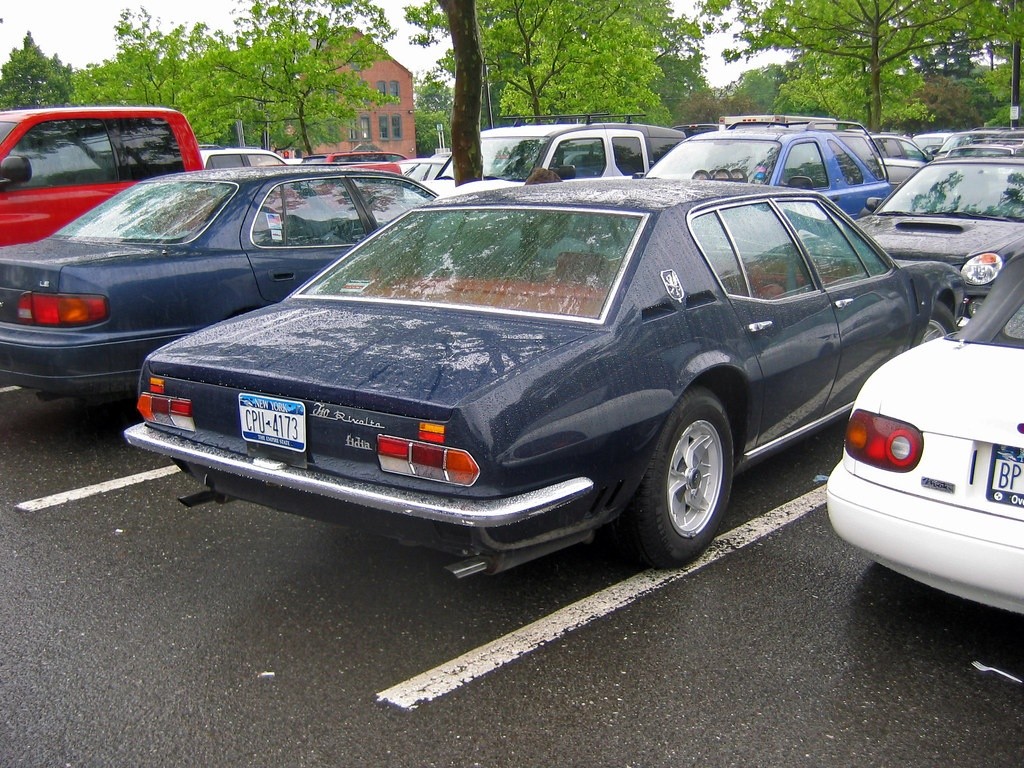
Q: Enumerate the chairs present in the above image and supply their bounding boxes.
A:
[285,208,348,244]
[556,249,607,284]
[746,262,785,299]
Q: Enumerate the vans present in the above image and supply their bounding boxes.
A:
[0,105,207,253]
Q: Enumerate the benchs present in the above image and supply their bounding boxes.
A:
[400,274,610,301]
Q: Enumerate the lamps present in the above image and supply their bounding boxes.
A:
[408,110,413,114]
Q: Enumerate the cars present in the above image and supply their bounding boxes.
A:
[815,154,1024,316]
[0,164,473,402]
[197,110,1024,287]
[826,249,1024,614]
[124,178,969,579]
